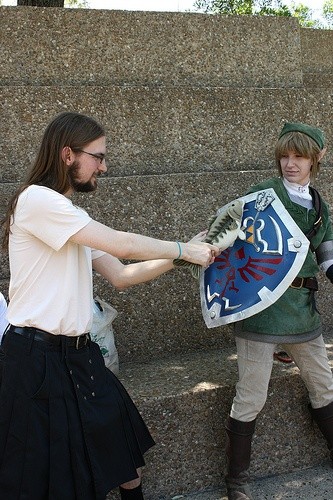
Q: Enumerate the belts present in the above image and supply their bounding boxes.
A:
[291,277,324,292]
[9,323,93,349]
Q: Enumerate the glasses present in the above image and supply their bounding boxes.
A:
[74,148,106,164]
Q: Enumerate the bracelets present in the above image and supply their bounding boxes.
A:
[175,240,182,261]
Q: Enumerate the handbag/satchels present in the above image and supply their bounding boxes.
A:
[89,296,120,379]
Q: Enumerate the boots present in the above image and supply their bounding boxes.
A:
[223,413,257,500]
[308,399,332,466]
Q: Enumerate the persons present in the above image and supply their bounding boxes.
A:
[200,122,333,500]
[0,113,219,500]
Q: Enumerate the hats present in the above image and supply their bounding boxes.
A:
[278,119,325,151]
